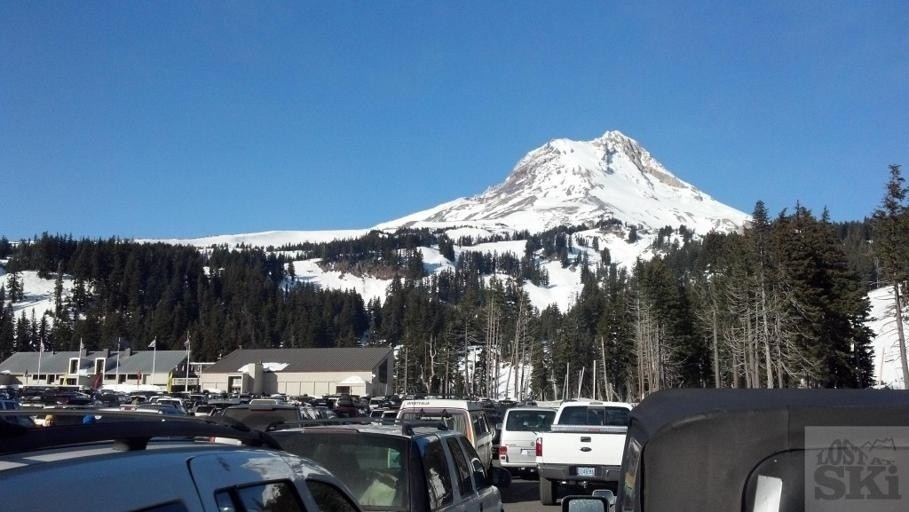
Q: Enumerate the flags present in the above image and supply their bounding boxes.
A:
[185,337,190,348]
[147,340,156,348]
[81,341,85,349]
[41,341,45,352]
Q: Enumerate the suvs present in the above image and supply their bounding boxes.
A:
[560,390,909,509]
